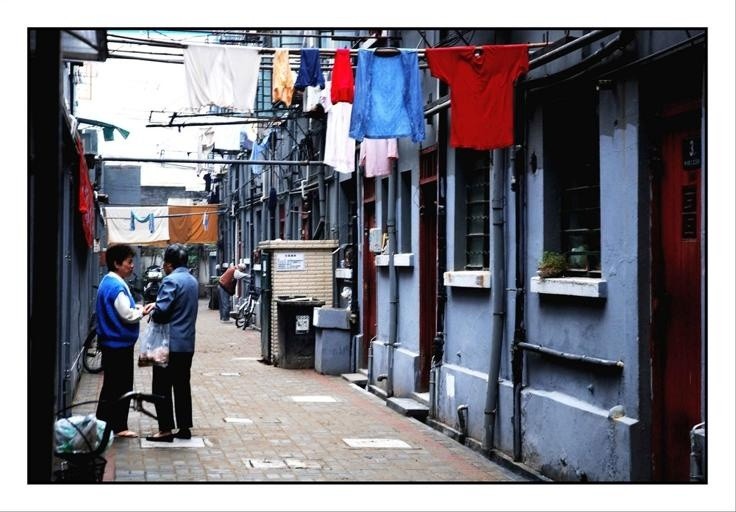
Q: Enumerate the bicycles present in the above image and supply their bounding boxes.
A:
[46,389,167,483]
[82,279,142,374]
[235,277,265,330]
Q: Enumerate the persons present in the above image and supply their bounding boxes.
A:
[215,262,251,324]
[93,242,153,439]
[144,242,200,443]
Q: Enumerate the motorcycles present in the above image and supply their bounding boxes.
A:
[141,264,164,308]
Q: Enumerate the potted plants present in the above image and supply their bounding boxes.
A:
[537,249,567,279]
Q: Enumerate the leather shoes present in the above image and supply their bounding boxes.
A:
[146,429,191,442]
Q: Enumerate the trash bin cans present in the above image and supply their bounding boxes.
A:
[273,296,325,369]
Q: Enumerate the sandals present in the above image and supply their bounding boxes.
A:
[114,430,137,437]
